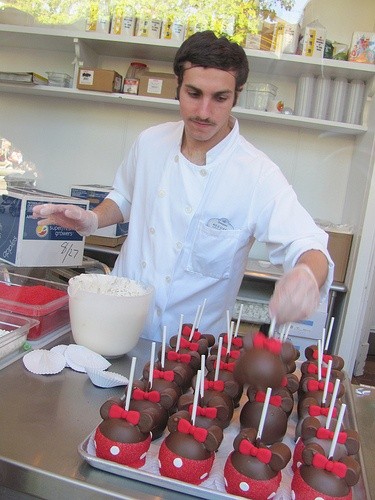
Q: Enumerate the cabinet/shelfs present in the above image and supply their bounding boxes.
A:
[0,7,375,383]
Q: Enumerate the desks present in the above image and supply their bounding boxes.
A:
[0,330,375,500]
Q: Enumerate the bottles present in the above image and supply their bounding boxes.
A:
[122,62,149,94]
[293,74,366,126]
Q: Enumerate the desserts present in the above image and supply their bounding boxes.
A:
[95,323,363,500]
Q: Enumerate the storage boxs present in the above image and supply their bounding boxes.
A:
[0,272,69,360]
[0,186,90,268]
[325,231,354,283]
[70,184,129,247]
[45,71,72,87]
[137,72,178,99]
[348,32,375,64]
[77,68,123,93]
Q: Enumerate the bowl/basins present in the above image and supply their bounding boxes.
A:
[237,82,278,112]
[67,275,155,360]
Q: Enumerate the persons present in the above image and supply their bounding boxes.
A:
[32,29,334,347]
